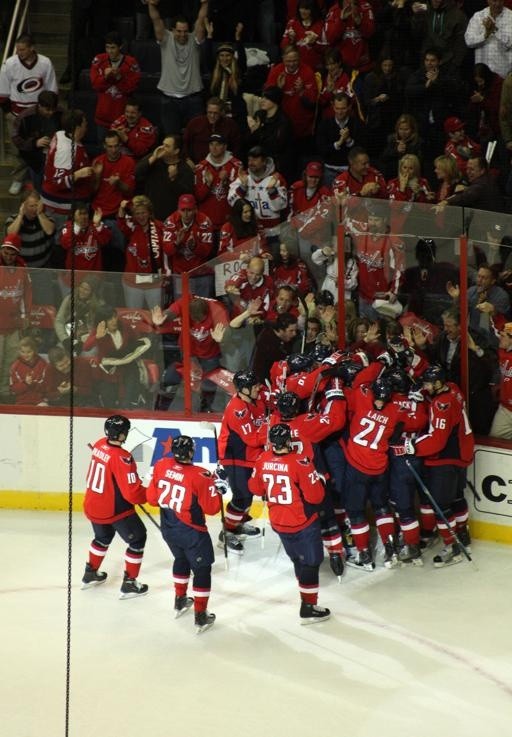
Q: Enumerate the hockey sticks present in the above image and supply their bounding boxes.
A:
[407,459,482,574]
[260,491,267,551]
[291,288,311,353]
[213,426,235,577]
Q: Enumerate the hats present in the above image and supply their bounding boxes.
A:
[2,234,21,252]
[444,117,463,130]
[177,194,195,210]
[306,162,322,178]
[208,131,223,143]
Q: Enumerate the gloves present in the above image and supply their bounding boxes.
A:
[212,469,227,494]
[324,377,345,401]
[322,350,351,365]
[377,351,393,367]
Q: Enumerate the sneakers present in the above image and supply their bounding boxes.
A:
[300,604,329,617]
[219,523,260,550]
[83,571,107,582]
[175,596,193,610]
[195,612,215,625]
[121,578,147,593]
[331,528,471,576]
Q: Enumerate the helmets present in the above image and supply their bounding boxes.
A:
[278,344,445,417]
[269,424,290,444]
[104,415,130,441]
[233,370,258,389]
[171,436,193,460]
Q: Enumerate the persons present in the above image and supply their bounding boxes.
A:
[217,340,475,625]
[146,436,229,634]
[80,414,149,600]
[1,1,512,443]
